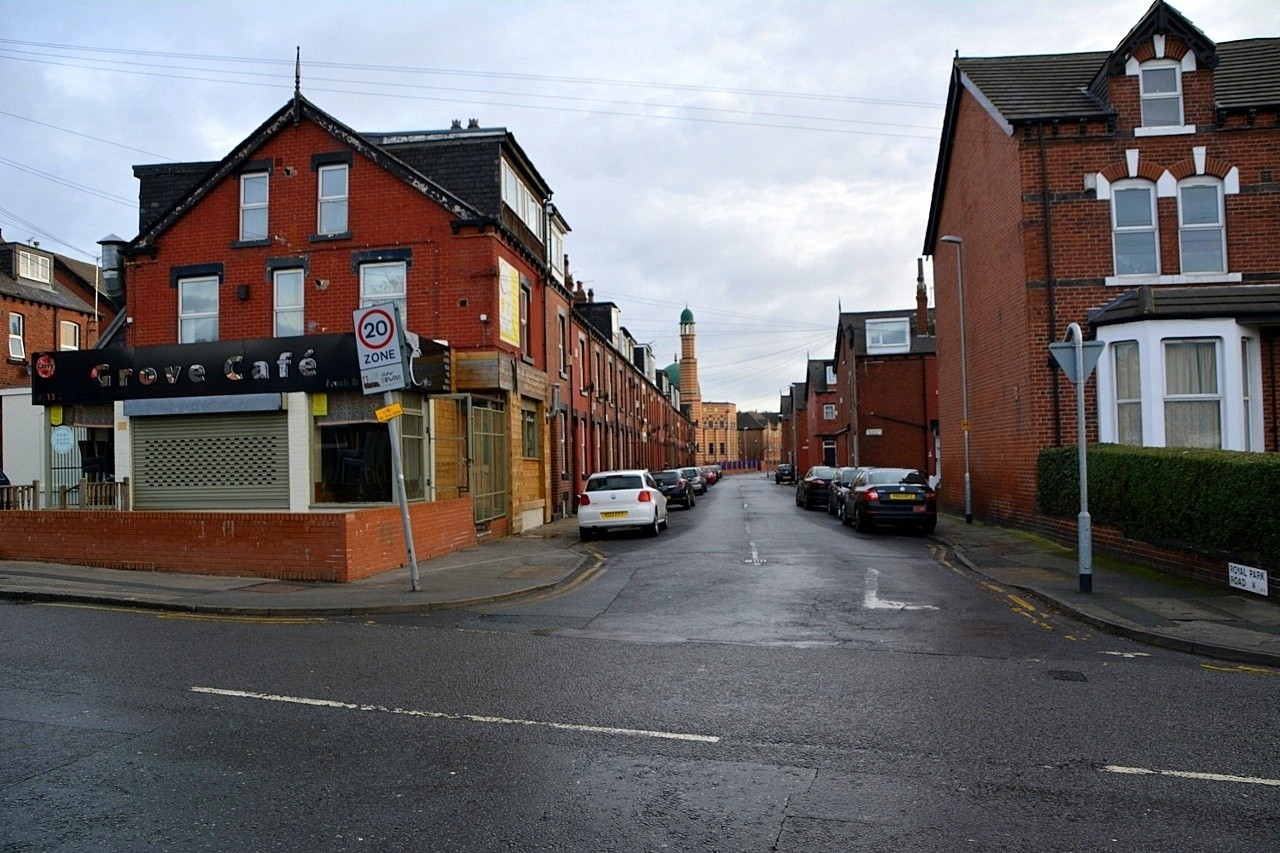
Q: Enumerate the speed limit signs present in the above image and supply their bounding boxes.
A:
[351,301,408,397]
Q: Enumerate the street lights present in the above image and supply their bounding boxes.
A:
[787,384,798,484]
[744,427,751,473]
[941,234,975,528]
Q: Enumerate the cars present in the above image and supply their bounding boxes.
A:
[702,466,719,485]
[825,466,871,521]
[775,464,796,485]
[576,468,670,538]
[679,466,709,496]
[796,466,840,510]
[650,469,696,509]
[711,465,724,479]
[841,467,939,534]
